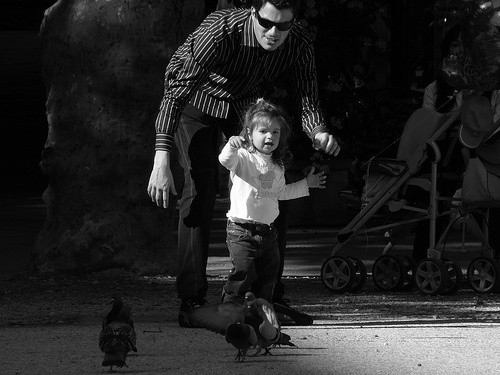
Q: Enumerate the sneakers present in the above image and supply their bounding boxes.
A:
[273,298,313,325]
[178,297,210,328]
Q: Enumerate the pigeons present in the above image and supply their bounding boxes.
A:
[99,306,137,372]
[102,293,138,353]
[246,301,297,357]
[185,292,282,350]
[224,319,258,362]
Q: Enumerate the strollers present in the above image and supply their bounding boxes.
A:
[321,101,500,295]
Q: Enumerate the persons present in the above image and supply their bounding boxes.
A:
[218,97,326,324]
[411,24,500,294]
[147,0,342,329]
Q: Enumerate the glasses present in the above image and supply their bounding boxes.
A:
[255,9,295,31]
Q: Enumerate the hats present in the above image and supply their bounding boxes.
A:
[458,95,493,149]
